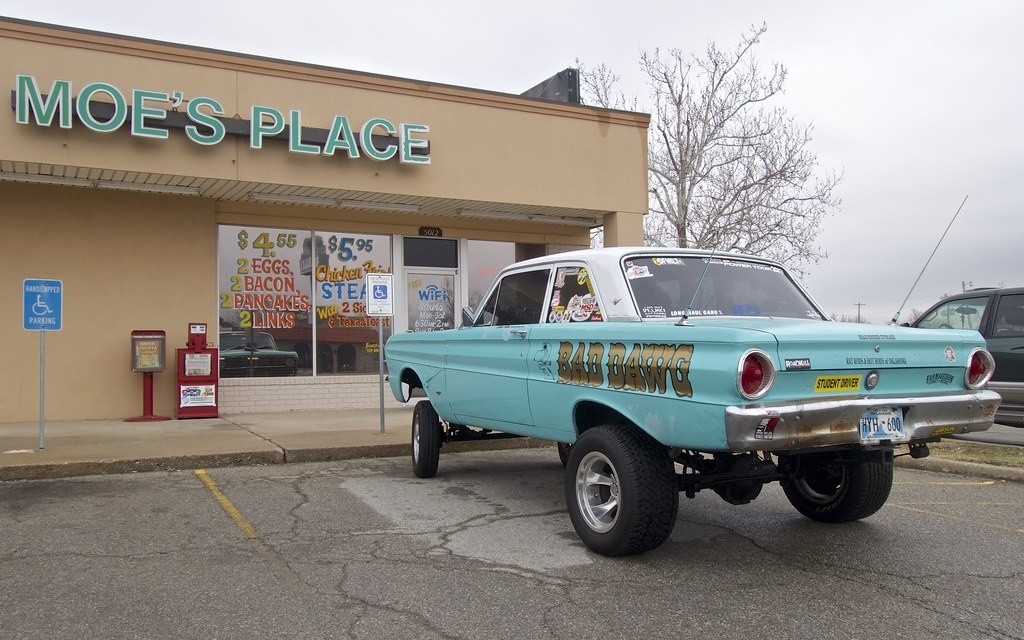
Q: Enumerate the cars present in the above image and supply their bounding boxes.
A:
[895,287,1024,429]
[385,246,1001,559]
[217,330,300,376]
